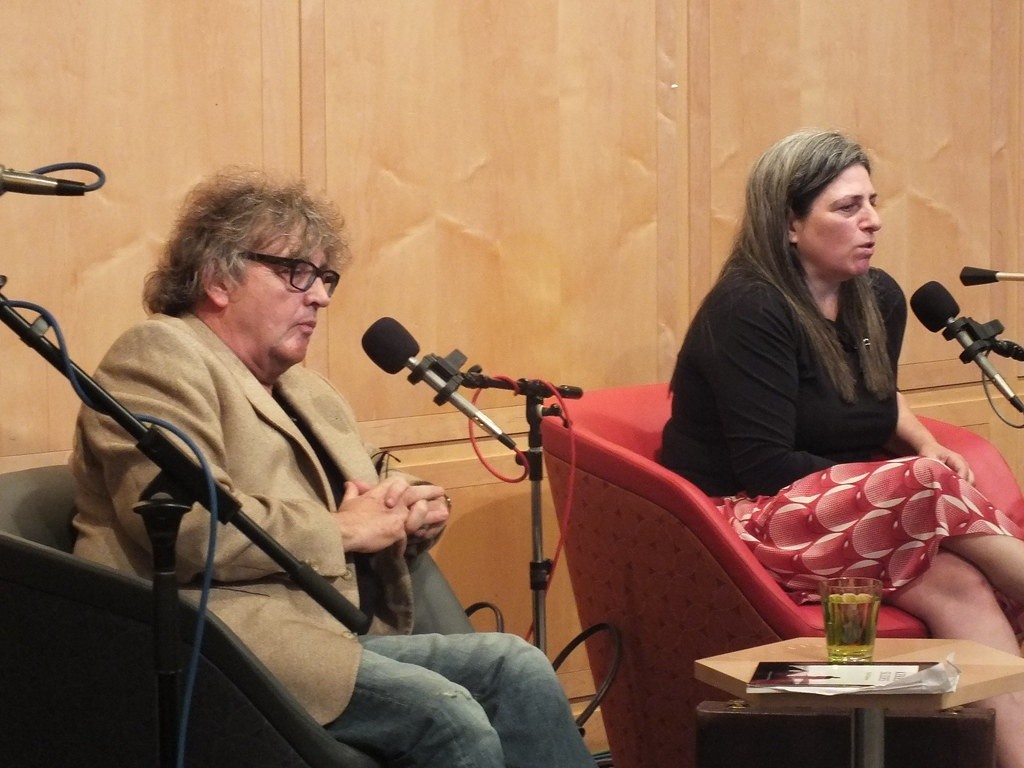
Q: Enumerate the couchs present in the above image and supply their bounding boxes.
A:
[541,388,1024,768]
[0,464,475,768]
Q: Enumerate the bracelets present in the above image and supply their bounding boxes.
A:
[444,495,452,509]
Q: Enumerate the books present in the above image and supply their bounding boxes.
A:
[746,661,942,693]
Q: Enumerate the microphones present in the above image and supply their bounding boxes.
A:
[909,281,1024,413]
[0,164,85,197]
[362,317,517,449]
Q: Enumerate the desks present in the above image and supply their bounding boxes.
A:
[693,636,1024,768]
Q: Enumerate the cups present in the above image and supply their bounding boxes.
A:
[819,577,882,662]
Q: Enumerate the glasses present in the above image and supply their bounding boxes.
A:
[238,253,340,298]
[371,450,401,479]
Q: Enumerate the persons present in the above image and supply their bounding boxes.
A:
[660,130,1024,768]
[69,165,600,768]
